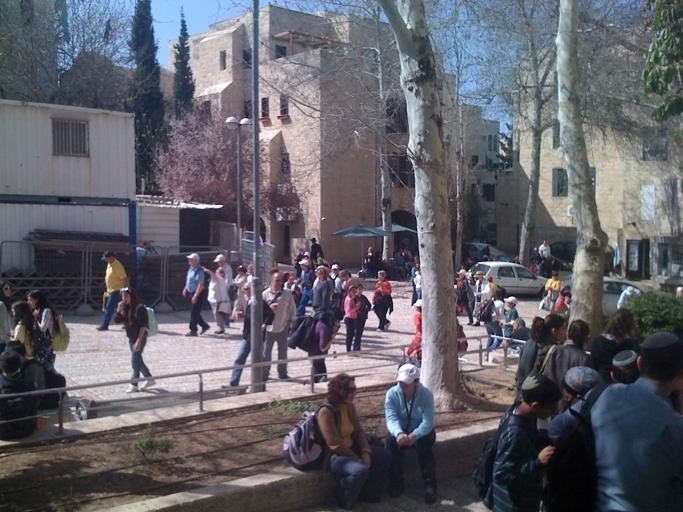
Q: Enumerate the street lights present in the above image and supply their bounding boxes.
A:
[225,117,251,254]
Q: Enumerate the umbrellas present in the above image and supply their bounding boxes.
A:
[372,224,418,233]
[328,223,391,278]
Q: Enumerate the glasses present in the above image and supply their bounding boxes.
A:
[346,387,356,393]
[5,287,12,290]
[122,287,131,293]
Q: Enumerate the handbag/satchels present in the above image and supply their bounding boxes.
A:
[102,292,117,313]
[218,302,231,314]
[352,435,392,476]
[227,285,237,300]
[332,291,341,308]
[542,380,611,512]
[551,291,558,300]
[539,296,551,311]
[473,415,537,499]
[292,287,302,304]
[536,253,542,262]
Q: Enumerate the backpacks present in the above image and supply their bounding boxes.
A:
[20,359,65,409]
[0,373,36,440]
[18,320,55,367]
[122,304,158,336]
[477,296,496,321]
[293,317,317,350]
[39,306,69,351]
[283,403,340,471]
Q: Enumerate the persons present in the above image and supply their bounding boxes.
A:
[181,252,211,337]
[113,285,156,394]
[371,270,394,332]
[315,372,393,511]
[461,236,560,268]
[406,269,529,364]
[362,241,419,269]
[383,364,443,504]
[287,304,343,384]
[212,253,234,327]
[481,369,563,511]
[353,285,370,351]
[513,313,572,402]
[535,269,571,313]
[25,290,56,373]
[607,346,640,386]
[587,307,644,373]
[4,339,48,411]
[7,301,42,363]
[540,319,590,392]
[343,284,361,351]
[261,266,294,383]
[0,278,17,357]
[559,365,602,415]
[282,235,351,304]
[0,349,40,441]
[93,250,131,331]
[229,264,248,322]
[200,265,231,334]
[220,280,274,392]
[547,332,682,512]
[246,262,254,277]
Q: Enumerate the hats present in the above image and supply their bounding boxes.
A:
[101,251,113,261]
[457,270,468,275]
[612,349,637,367]
[504,296,517,304]
[625,286,634,295]
[298,259,309,266]
[565,366,601,395]
[186,253,199,261]
[413,299,422,307]
[315,265,324,272]
[304,252,310,258]
[396,363,420,385]
[473,271,483,277]
[213,254,225,263]
[522,370,561,403]
[551,271,559,276]
[639,332,683,360]
[332,264,339,269]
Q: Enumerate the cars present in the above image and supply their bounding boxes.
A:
[602,278,645,315]
[464,261,548,299]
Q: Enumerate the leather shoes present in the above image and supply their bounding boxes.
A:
[200,325,209,334]
[185,332,197,336]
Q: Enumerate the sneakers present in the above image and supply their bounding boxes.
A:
[485,355,488,360]
[223,325,229,328]
[424,480,436,505]
[327,498,352,512]
[126,383,137,392]
[376,329,384,332]
[281,376,292,381]
[140,379,155,389]
[214,328,224,334]
[472,322,479,326]
[386,321,391,329]
[221,383,231,388]
